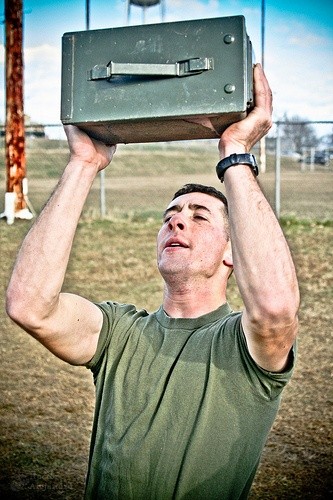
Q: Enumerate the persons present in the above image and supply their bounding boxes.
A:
[6,62,302,500]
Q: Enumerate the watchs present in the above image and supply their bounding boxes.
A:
[216,153,258,183]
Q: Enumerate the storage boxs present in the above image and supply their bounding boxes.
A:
[59,15,255,145]
